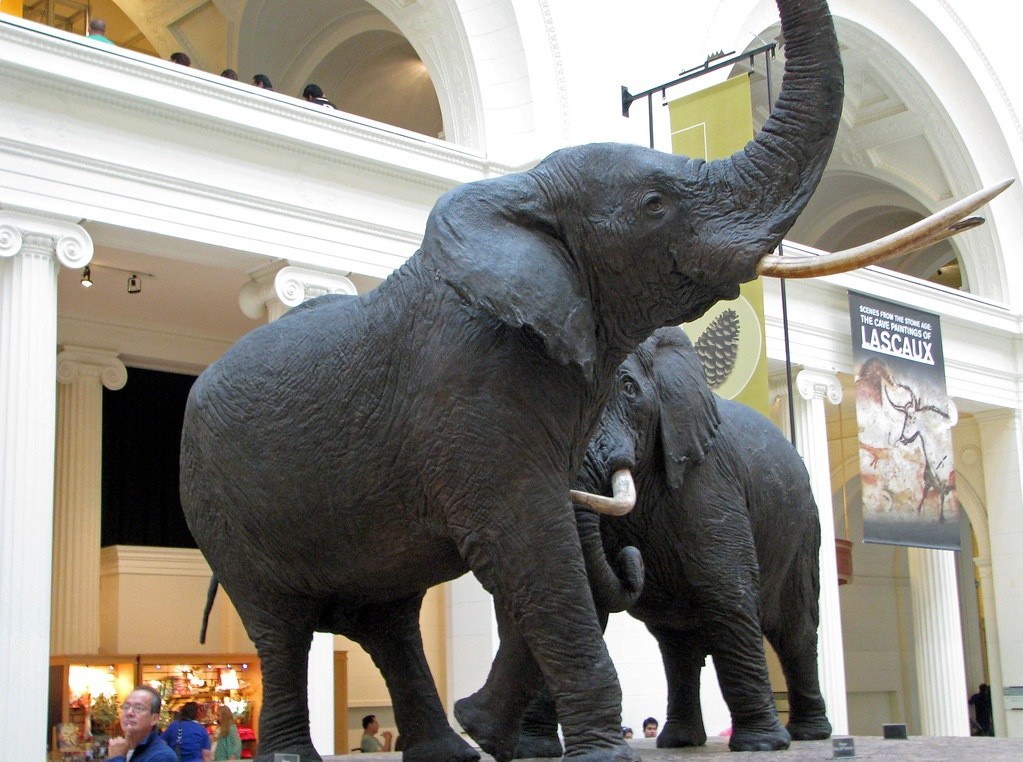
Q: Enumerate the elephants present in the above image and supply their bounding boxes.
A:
[180,0,1015,762]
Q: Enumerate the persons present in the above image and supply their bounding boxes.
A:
[84,18,114,44]
[213,705,242,762]
[170,52,191,67]
[968,684,993,736]
[221,69,239,81]
[302,84,338,110]
[360,715,392,752]
[159,700,213,762]
[108,685,178,762]
[622,726,633,739]
[252,75,273,90]
[643,717,658,738]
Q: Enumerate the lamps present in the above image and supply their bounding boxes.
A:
[127,274,141,294]
[80,266,93,288]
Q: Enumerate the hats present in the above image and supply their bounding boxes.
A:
[622,726,633,736]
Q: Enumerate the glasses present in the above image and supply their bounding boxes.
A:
[120,704,152,713]
